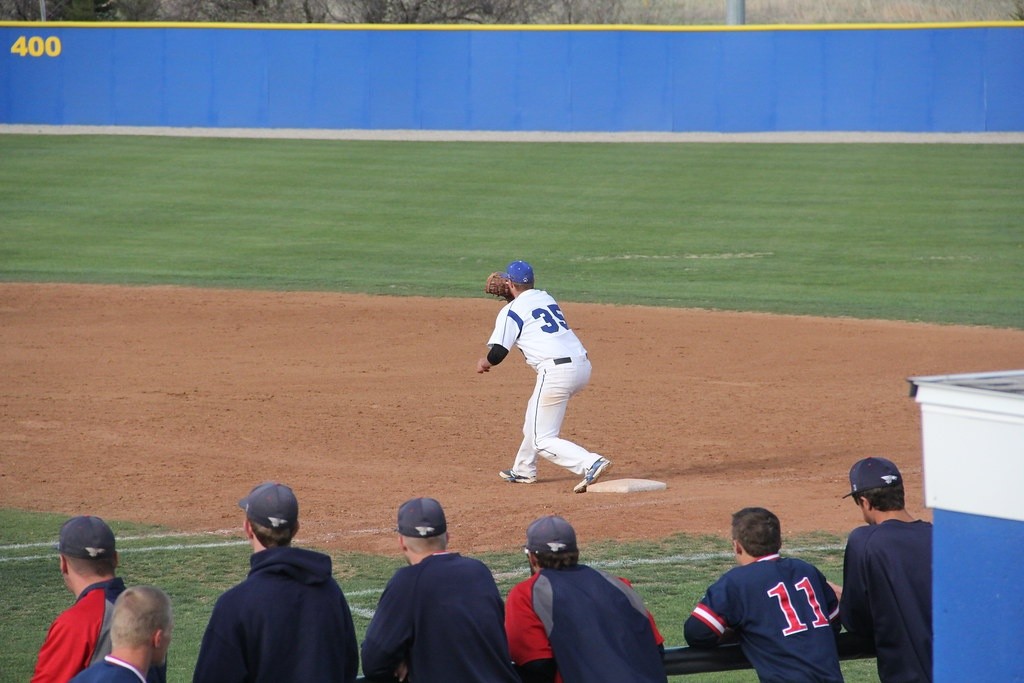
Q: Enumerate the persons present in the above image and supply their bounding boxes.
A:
[684,506,848,682]
[67,584,173,683]
[359,493,528,683]
[504,514,670,683]
[824,457,935,683]
[32,514,168,683]
[477,261,615,496]
[194,479,361,683]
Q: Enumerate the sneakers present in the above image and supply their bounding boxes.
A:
[574,457,614,493]
[499,470,537,485]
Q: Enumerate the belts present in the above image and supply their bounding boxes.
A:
[553,354,588,365]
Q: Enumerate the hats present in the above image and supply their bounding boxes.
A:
[500,260,535,285]
[238,482,298,527]
[394,496,448,538]
[524,515,577,553]
[842,457,904,501]
[55,516,116,558]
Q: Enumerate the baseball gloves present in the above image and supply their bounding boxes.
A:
[485,272,514,304]
